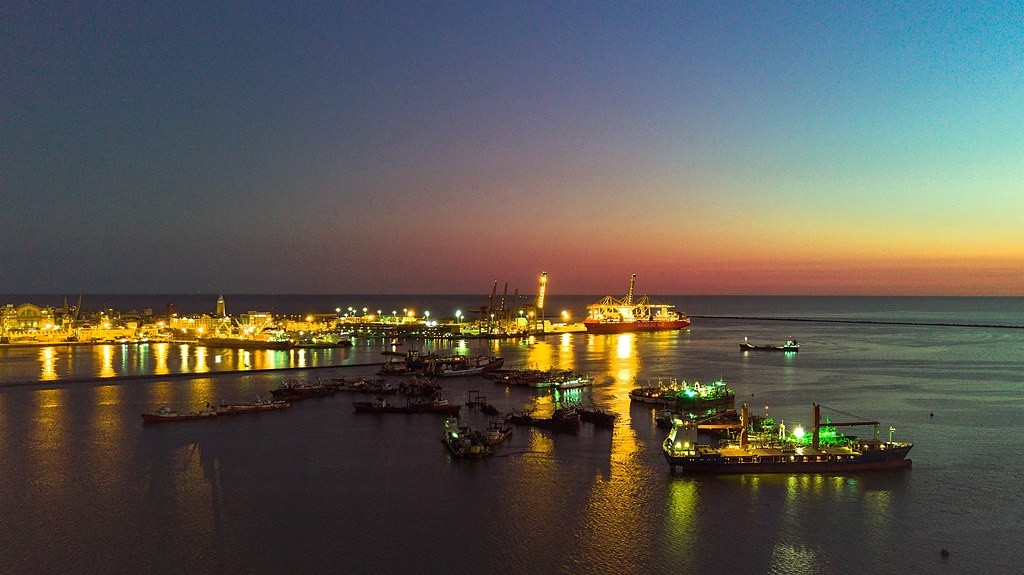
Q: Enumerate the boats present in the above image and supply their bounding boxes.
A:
[352,393,464,416]
[139,403,218,422]
[506,401,582,433]
[661,403,915,473]
[574,396,615,426]
[739,336,802,353]
[333,377,346,387]
[480,403,500,416]
[380,350,595,412]
[196,336,297,350]
[481,416,512,444]
[440,414,494,459]
[651,407,779,434]
[627,379,737,408]
[581,274,693,334]
[346,375,398,394]
[268,376,338,399]
[209,397,291,414]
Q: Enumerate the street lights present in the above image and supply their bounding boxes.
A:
[424,311,430,321]
[519,310,524,318]
[392,311,396,321]
[490,314,494,324]
[403,308,407,319]
[336,307,341,318]
[346,306,381,319]
[460,316,464,326]
